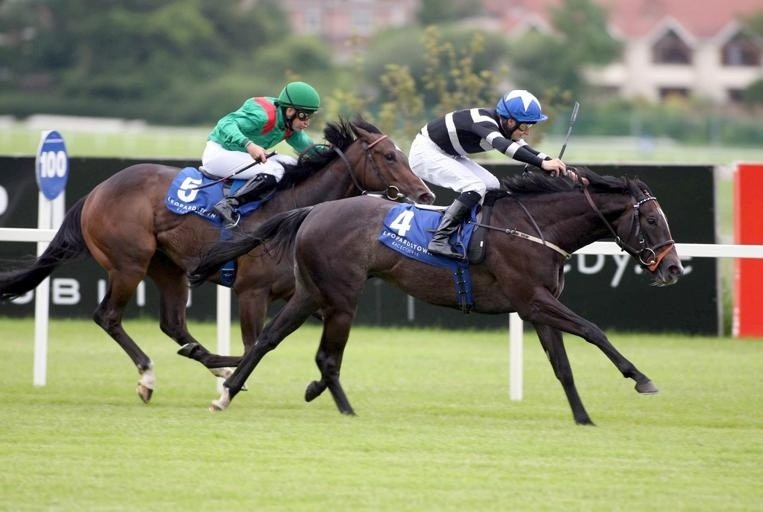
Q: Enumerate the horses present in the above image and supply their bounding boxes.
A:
[182,167,684,428]
[0,109,438,414]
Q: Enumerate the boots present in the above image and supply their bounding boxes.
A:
[213,173,277,232]
[427,199,468,259]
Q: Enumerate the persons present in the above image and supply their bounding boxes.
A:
[408,87,568,262]
[201,80,334,232]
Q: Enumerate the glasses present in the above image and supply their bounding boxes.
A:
[518,122,536,131]
[295,111,313,121]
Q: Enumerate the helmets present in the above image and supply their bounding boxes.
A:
[278,81,320,110]
[495,90,549,122]
